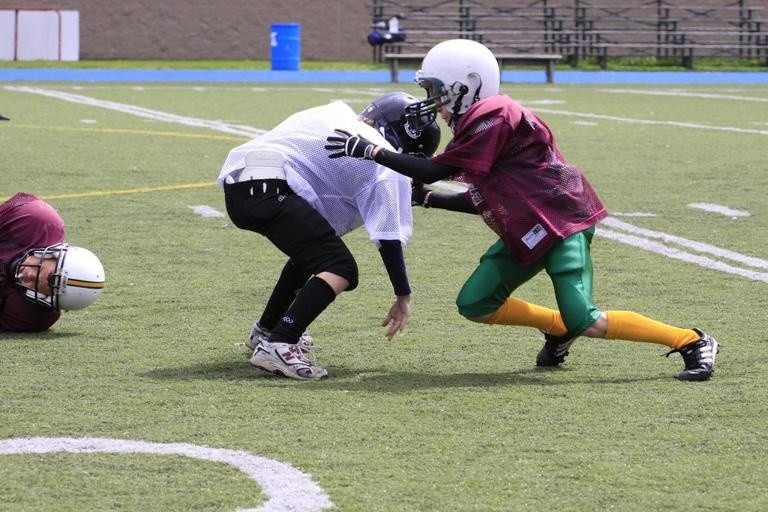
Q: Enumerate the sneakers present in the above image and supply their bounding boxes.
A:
[244,323,313,352]
[665,327,719,381]
[248,336,328,381]
[536,328,576,367]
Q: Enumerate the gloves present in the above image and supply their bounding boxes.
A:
[324,128,378,160]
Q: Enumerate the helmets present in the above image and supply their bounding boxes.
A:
[399,38,500,140]
[8,242,105,312]
[359,90,440,158]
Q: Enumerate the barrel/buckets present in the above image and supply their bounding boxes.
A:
[269,23,301,71]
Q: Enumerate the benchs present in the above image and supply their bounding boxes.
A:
[371,0,768,82]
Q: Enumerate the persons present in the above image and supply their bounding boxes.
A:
[324,38,719,381]
[215,90,441,380]
[0,191,107,333]
